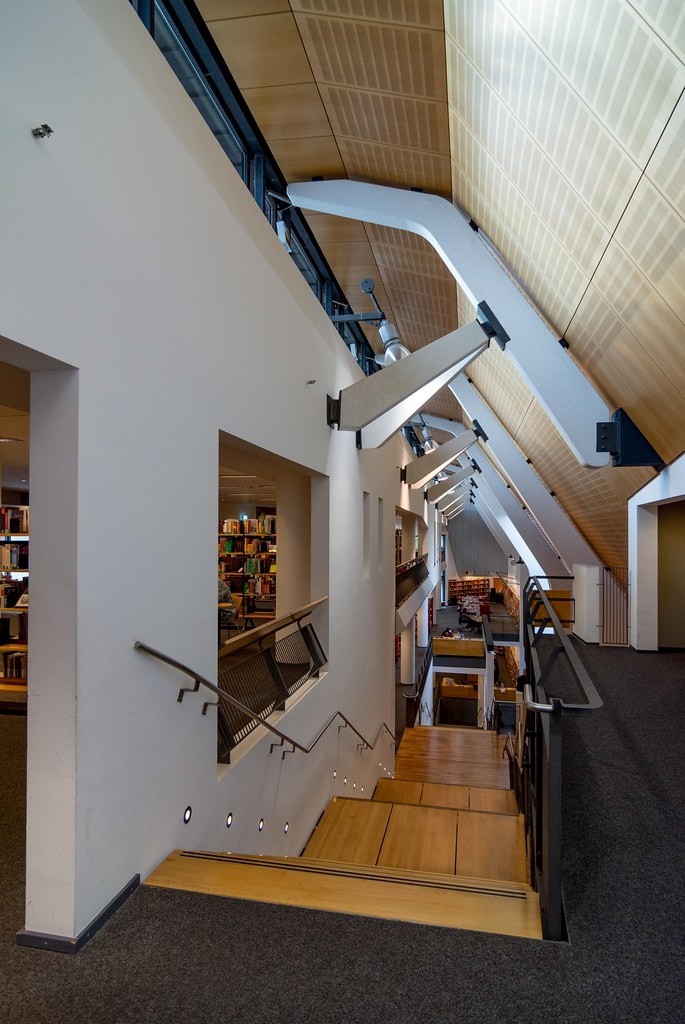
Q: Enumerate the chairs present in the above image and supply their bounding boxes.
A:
[218,595,243,639]
[456,608,480,633]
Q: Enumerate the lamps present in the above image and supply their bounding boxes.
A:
[496,571,520,587]
[329,279,411,367]
[515,556,524,565]
[434,472,448,482]
[404,413,440,455]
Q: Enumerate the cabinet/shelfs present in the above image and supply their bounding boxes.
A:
[502,583,520,628]
[395,633,401,658]
[218,533,277,630]
[503,646,519,683]
[448,579,490,604]
[429,596,435,630]
[0,529,30,691]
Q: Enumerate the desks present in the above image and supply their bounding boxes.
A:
[432,636,484,657]
[494,687,516,700]
[479,600,490,621]
[218,602,233,648]
[442,684,478,699]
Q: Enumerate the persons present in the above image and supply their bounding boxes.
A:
[461,608,470,629]
[495,680,504,687]
[516,668,527,692]
[453,629,464,638]
[441,628,453,637]
[218,578,237,622]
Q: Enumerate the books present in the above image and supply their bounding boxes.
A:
[0,505,29,679]
[218,515,276,615]
